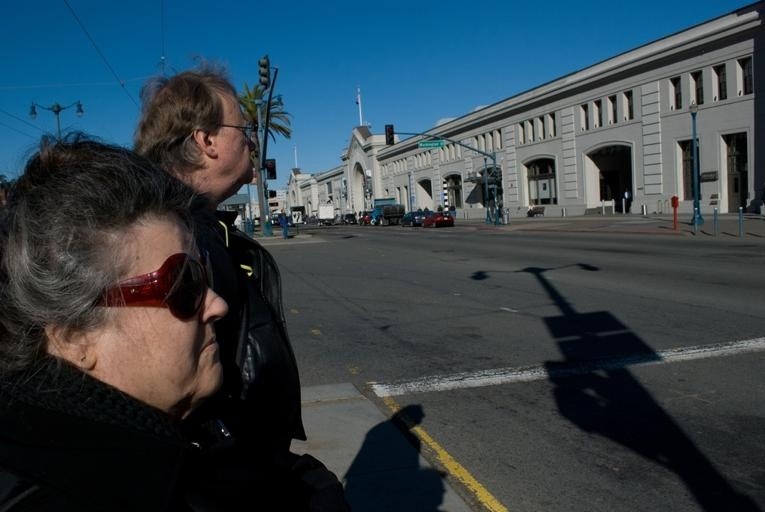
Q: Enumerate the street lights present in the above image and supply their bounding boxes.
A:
[688,98,705,226]
[385,124,395,147]
[24,100,86,140]
[483,153,493,225]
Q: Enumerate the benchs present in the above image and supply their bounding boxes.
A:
[533,206,546,216]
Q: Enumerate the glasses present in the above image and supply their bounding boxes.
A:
[92,247,216,320]
[219,118,257,142]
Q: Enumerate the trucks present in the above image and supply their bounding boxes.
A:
[317,202,336,227]
[369,197,405,226]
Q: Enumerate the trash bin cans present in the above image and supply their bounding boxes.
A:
[264,222,273,236]
[244,220,254,236]
[643,204,647,216]
[501,207,510,225]
[562,207,566,218]
[760,207,765,215]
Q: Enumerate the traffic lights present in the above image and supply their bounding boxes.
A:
[267,158,277,180]
[269,190,276,198]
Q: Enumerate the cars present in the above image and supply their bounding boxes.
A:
[358,210,375,225]
[400,210,428,227]
[252,204,306,228]
[342,213,357,224]
[422,210,456,229]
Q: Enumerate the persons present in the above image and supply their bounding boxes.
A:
[266,204,460,240]
[528,201,535,218]
[0,133,350,510]
[127,72,297,453]
[496,199,505,221]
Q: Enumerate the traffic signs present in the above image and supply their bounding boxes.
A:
[417,140,446,148]
